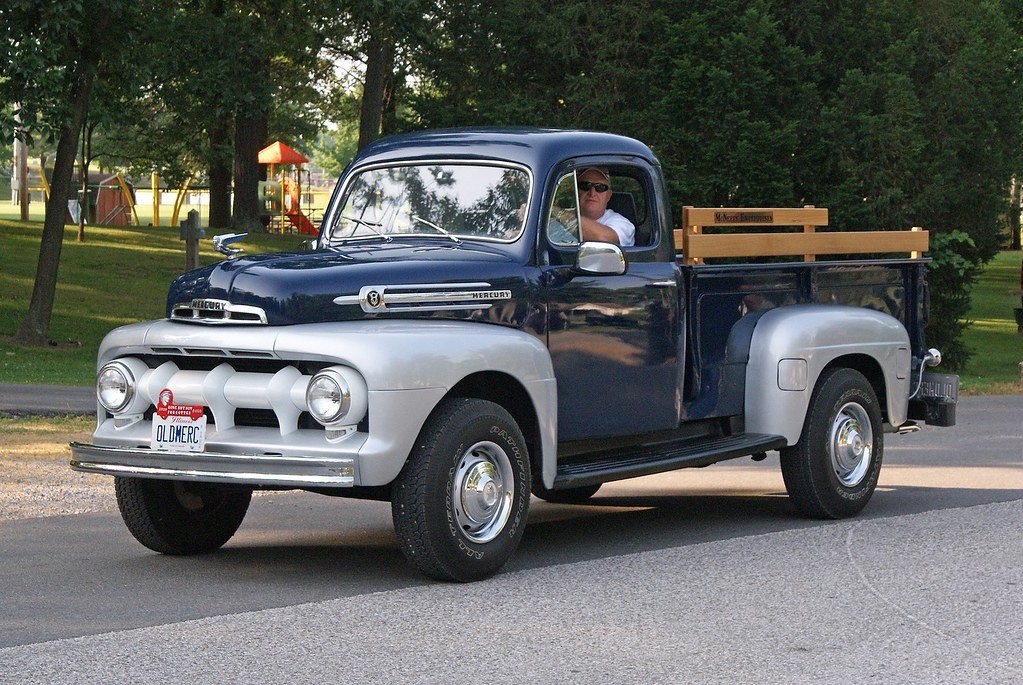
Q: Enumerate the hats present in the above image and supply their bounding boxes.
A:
[576,166,611,184]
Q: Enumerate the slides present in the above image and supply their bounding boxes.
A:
[286,208,318,236]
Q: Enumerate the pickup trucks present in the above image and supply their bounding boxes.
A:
[63,124,963,586]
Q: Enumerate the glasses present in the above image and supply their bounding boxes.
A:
[577,182,610,193]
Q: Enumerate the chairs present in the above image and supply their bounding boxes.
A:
[607,190,642,246]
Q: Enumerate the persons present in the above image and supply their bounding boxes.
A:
[496,165,636,247]
